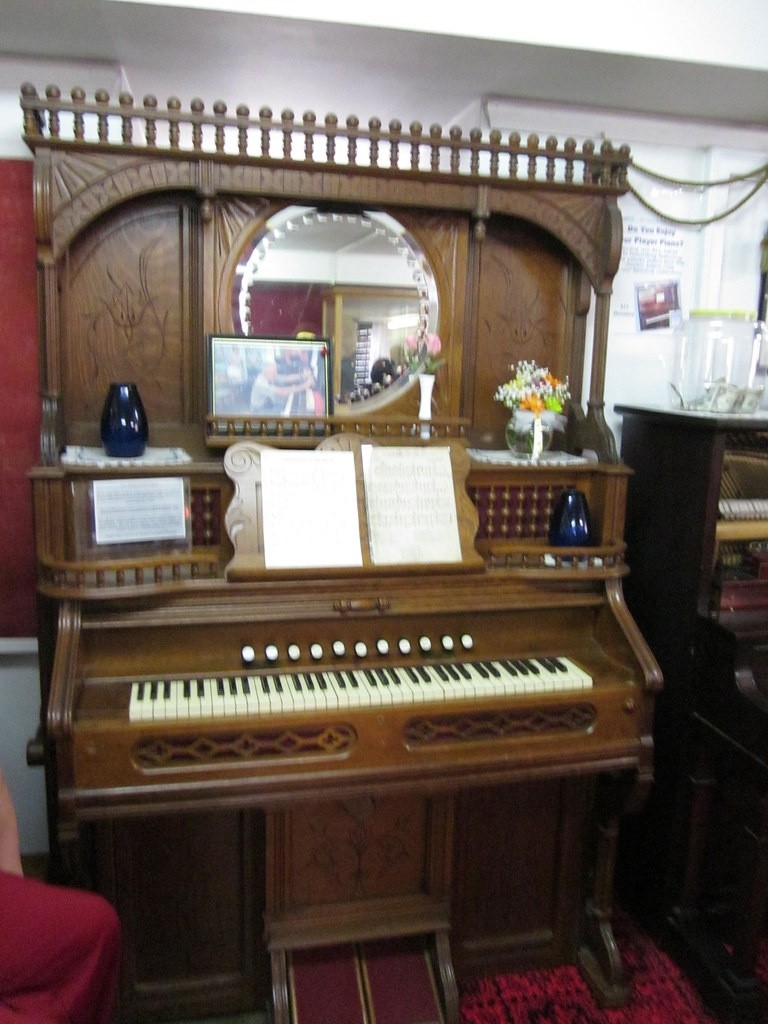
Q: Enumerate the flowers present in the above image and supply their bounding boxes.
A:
[493,359,571,412]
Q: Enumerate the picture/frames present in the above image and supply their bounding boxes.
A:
[203,335,332,437]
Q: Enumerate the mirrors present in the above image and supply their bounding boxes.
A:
[213,198,469,429]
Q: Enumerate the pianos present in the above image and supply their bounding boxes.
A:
[25,452,665,1024]
[610,400,768,1023]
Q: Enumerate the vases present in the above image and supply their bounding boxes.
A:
[548,489,591,559]
[98,382,146,459]
[505,410,553,456]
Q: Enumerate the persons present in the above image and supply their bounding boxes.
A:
[370,358,398,384]
[249,344,316,420]
[341,353,356,395]
